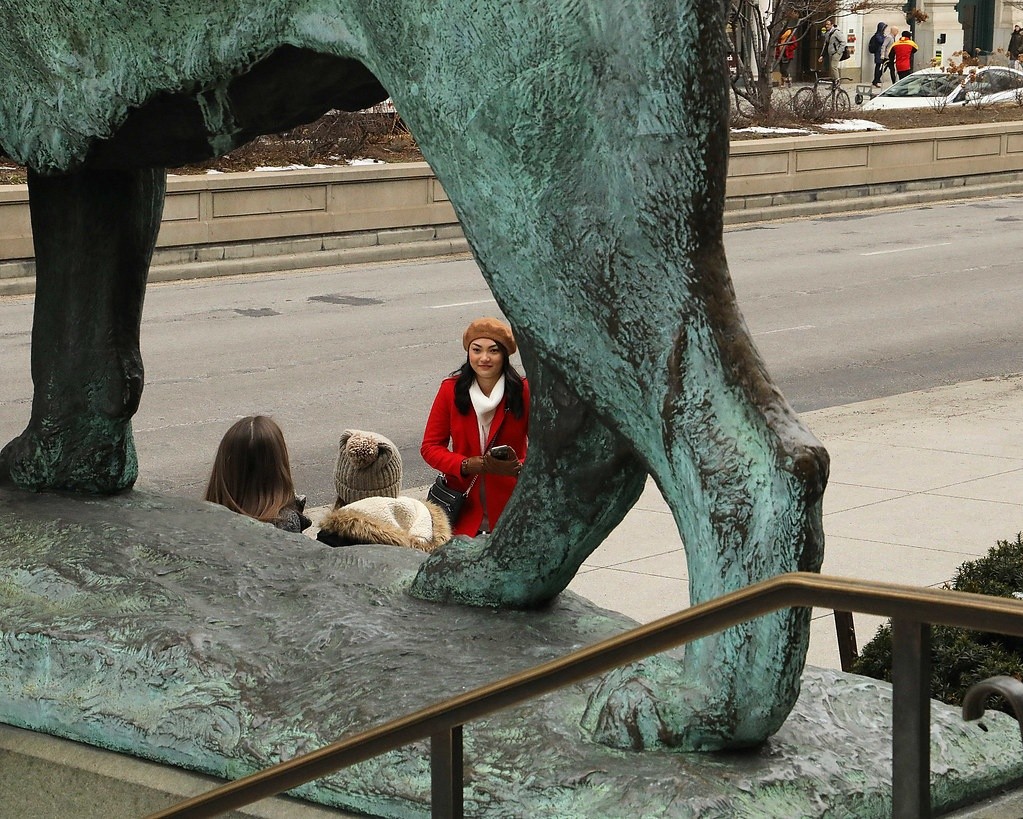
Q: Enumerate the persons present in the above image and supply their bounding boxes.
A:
[202,415,314,535]
[772,17,1023,92]
[420,319,531,538]
[315,432,452,552]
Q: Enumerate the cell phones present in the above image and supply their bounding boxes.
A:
[491,444,509,461]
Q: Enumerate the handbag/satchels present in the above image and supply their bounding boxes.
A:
[839,46,850,61]
[427,473,466,529]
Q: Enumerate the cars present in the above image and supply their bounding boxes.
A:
[858,65,1022,112]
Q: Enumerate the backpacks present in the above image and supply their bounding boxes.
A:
[868,35,878,54]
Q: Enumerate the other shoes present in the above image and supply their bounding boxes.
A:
[873,82,881,88]
[825,85,833,90]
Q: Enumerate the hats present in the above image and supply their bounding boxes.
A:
[1015,22,1022,29]
[334,428,403,504]
[462,317,516,357]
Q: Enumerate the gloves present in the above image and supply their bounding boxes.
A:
[461,451,523,476]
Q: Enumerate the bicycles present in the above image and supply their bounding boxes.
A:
[791,69,853,115]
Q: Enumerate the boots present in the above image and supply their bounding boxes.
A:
[785,77,792,87]
[780,77,786,87]
